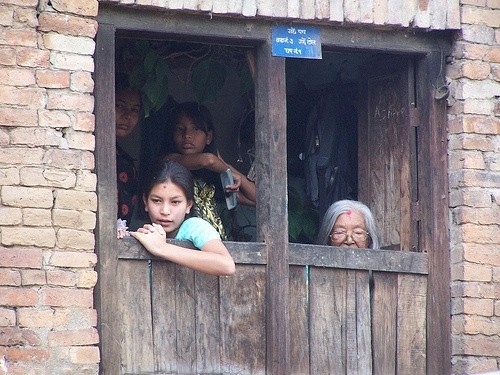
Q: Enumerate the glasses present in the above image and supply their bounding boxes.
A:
[330,229,369,241]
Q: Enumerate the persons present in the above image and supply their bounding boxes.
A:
[316,198,381,249]
[116,159,236,277]
[160,100,258,242]
[115,72,144,241]
